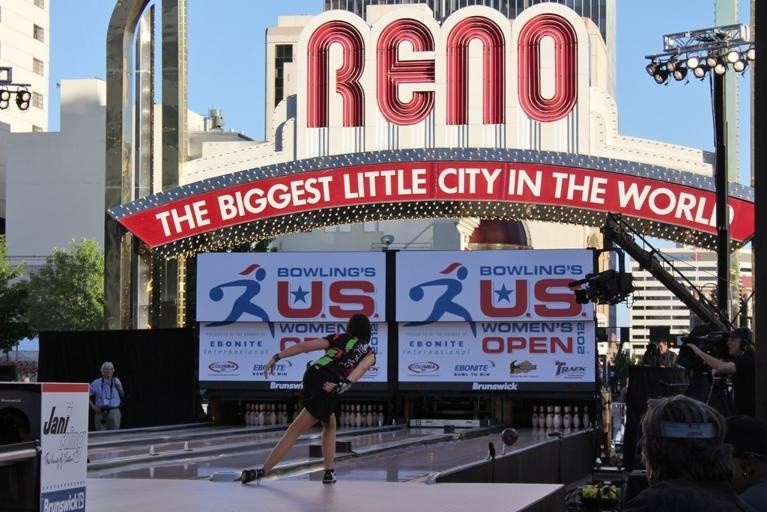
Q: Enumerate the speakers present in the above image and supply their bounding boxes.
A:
[0,380,41,511]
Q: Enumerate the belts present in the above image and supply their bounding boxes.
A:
[101,406,119,410]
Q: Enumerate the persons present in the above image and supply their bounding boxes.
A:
[238,312,377,484]
[87,361,126,430]
[681,325,754,416]
[642,336,677,368]
[714,413,766,512]
[606,392,762,511]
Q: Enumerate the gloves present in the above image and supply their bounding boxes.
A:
[330,379,351,396]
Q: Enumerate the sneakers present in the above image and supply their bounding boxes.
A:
[322,468,337,483]
[241,469,264,484]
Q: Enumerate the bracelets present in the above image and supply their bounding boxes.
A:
[272,353,280,362]
[119,388,123,392]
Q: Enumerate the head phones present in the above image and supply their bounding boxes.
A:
[739,330,751,352]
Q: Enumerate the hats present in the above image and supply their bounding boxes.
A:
[721,326,752,338]
[723,413,767,450]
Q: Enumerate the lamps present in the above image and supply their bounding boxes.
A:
[0,90,31,110]
[645,44,755,85]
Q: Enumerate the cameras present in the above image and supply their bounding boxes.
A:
[99,404,110,424]
